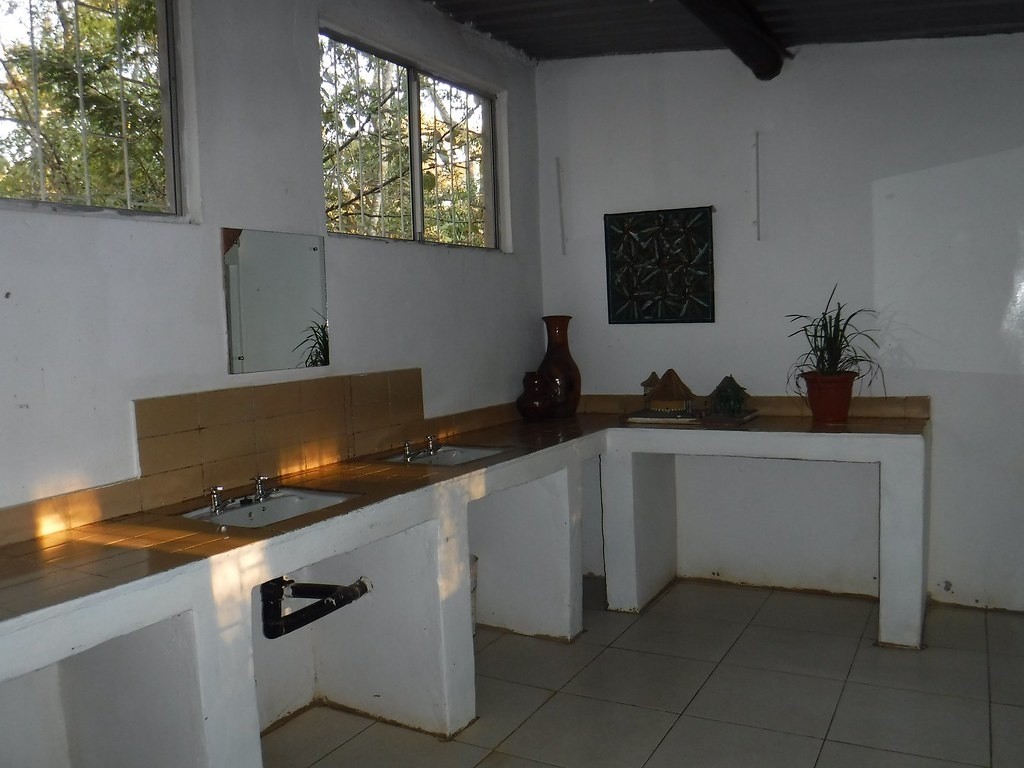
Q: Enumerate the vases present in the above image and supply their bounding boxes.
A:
[535,313,582,417]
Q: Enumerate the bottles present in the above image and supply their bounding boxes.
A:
[516,371,548,421]
[536,316,579,418]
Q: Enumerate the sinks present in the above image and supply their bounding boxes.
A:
[182,487,361,528]
[384,445,504,465]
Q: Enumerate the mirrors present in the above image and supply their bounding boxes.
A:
[219,227,330,376]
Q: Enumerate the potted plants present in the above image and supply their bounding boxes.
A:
[783,282,889,427]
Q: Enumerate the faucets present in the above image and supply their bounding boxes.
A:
[425,435,449,455]
[249,472,279,503]
[400,440,428,463]
[203,484,235,516]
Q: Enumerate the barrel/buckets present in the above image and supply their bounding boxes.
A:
[468,552,481,636]
